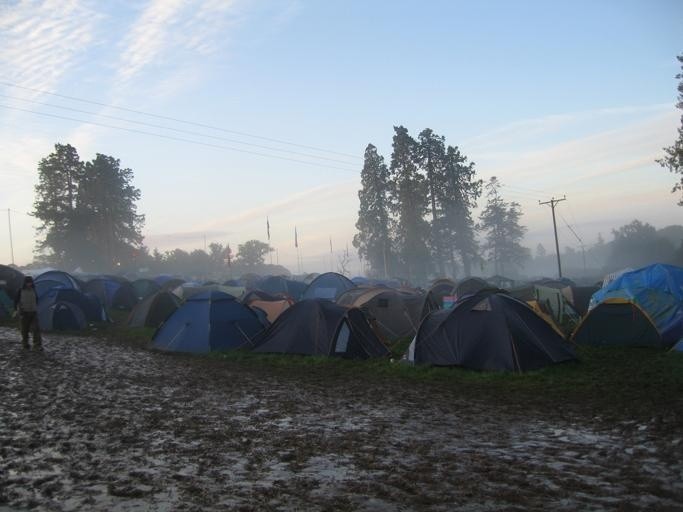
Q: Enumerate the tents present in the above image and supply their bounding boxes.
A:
[0,260,683,377]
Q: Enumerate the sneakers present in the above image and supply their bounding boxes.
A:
[24,344,43,352]
